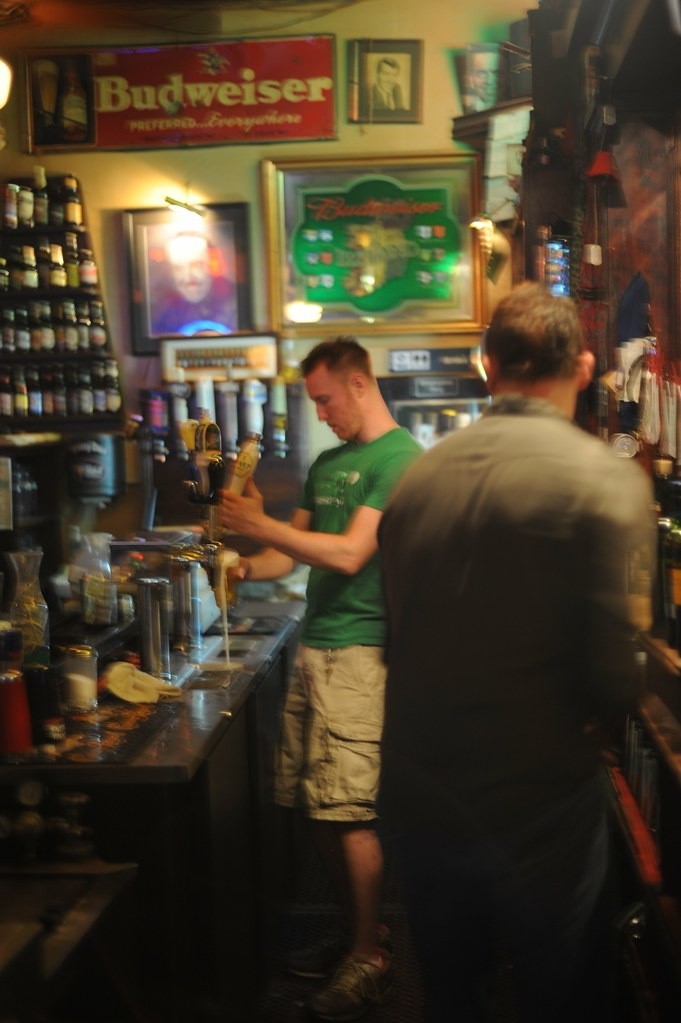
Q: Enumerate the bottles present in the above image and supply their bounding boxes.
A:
[0,165,120,415]
[654,459,681,653]
[148,378,289,528]
[7,551,51,665]
[79,533,117,624]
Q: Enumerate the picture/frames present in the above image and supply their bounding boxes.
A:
[346,37,424,124]
[259,151,489,339]
[158,331,282,383]
[17,33,340,155]
[122,202,257,356]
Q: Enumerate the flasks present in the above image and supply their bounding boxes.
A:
[139,579,173,681]
[169,562,203,647]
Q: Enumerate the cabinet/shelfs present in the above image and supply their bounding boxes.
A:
[0,172,128,425]
[613,633,681,974]
[0,654,294,1021]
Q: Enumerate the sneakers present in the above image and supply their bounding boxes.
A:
[287,924,393,978]
[310,933,414,1021]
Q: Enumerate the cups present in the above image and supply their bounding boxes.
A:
[23,665,55,742]
[0,669,31,753]
[60,645,98,711]
[207,547,239,610]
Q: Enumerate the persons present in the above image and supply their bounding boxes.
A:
[218,334,413,1000]
[375,281,658,1023]
[147,232,235,335]
